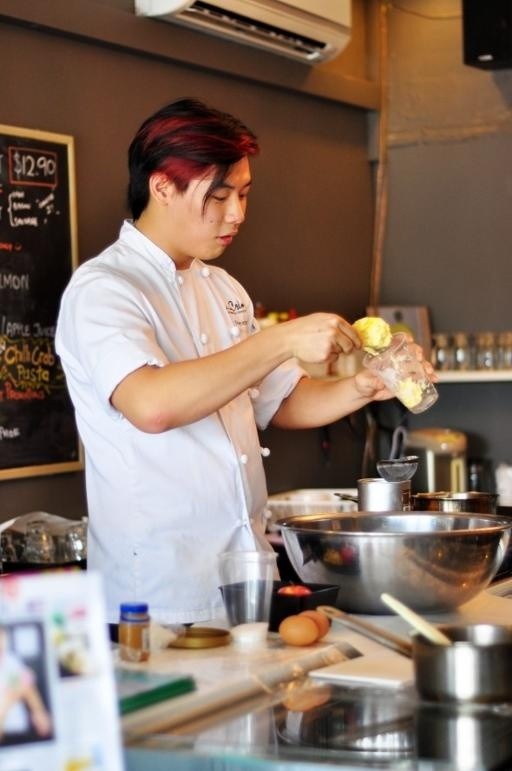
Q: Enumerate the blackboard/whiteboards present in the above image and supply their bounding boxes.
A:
[0,122,86,483]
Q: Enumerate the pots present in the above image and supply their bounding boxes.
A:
[316,604,512,701]
[325,708,512,770]
[334,479,498,512]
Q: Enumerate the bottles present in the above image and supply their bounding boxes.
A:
[117,603,152,660]
[432,332,511,369]
[469,457,495,490]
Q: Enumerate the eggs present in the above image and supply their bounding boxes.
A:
[301,609,331,639]
[279,614,318,646]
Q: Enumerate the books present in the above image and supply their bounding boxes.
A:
[115,664,194,716]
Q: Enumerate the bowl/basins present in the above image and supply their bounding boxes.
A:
[278,687,417,745]
[218,580,339,634]
[273,512,512,617]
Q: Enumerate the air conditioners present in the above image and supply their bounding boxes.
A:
[134,0,353,67]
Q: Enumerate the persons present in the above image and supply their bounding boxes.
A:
[52,96,442,647]
[2,624,52,749]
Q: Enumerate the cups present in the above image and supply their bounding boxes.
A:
[0,520,86,563]
[362,329,440,417]
[219,551,277,645]
